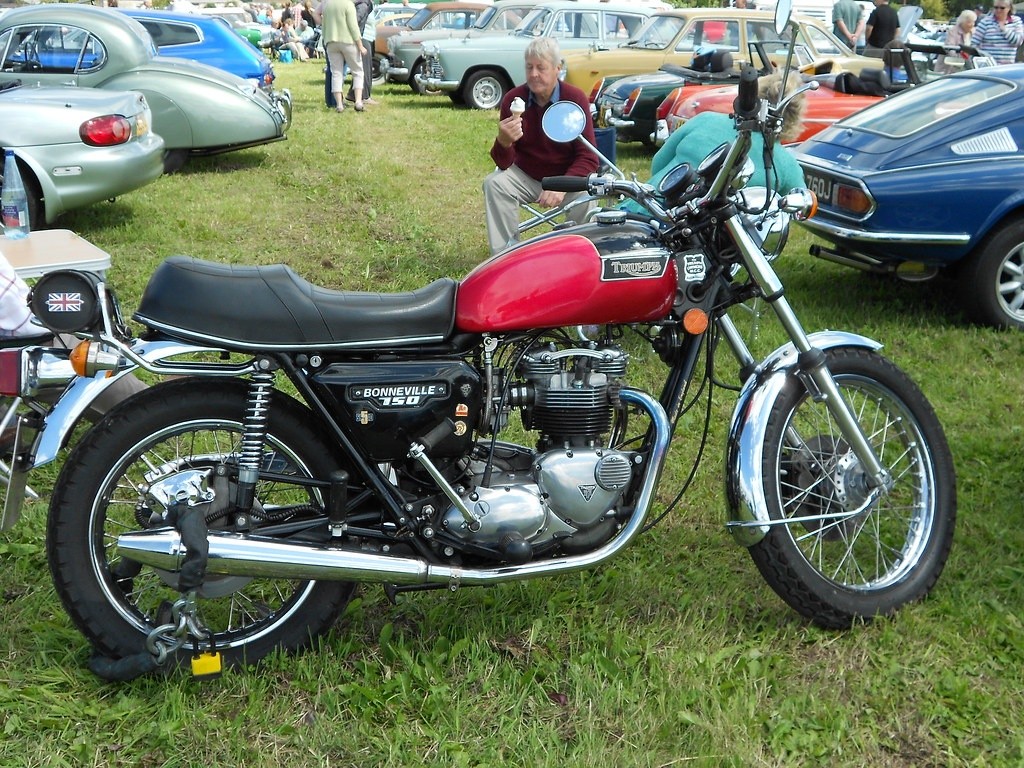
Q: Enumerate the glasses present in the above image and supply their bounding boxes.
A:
[994,6,1005,9]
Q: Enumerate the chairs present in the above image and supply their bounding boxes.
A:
[857,69,893,97]
[519,126,617,233]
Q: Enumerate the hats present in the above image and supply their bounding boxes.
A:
[975,4,984,11]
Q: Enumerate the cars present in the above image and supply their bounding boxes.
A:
[0,4,295,177]
[0,81,166,230]
[355,0,879,153]
[786,59,1024,333]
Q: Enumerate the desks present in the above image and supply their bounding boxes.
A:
[0,229,112,287]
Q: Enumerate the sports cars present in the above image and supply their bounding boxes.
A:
[651,39,1006,161]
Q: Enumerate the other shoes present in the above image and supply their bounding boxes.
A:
[355,105,363,111]
[337,105,346,113]
[363,97,378,104]
[345,94,356,102]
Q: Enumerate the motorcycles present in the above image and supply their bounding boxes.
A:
[0,0,960,695]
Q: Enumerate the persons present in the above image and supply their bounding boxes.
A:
[974,5,985,27]
[483,38,599,258]
[313,0,379,113]
[935,9,977,74]
[862,0,901,56]
[617,74,807,218]
[831,0,865,49]
[971,0,1024,65]
[246,0,316,63]
[728,0,753,44]
[144,0,152,9]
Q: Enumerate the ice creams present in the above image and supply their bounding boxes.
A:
[509,96,525,119]
